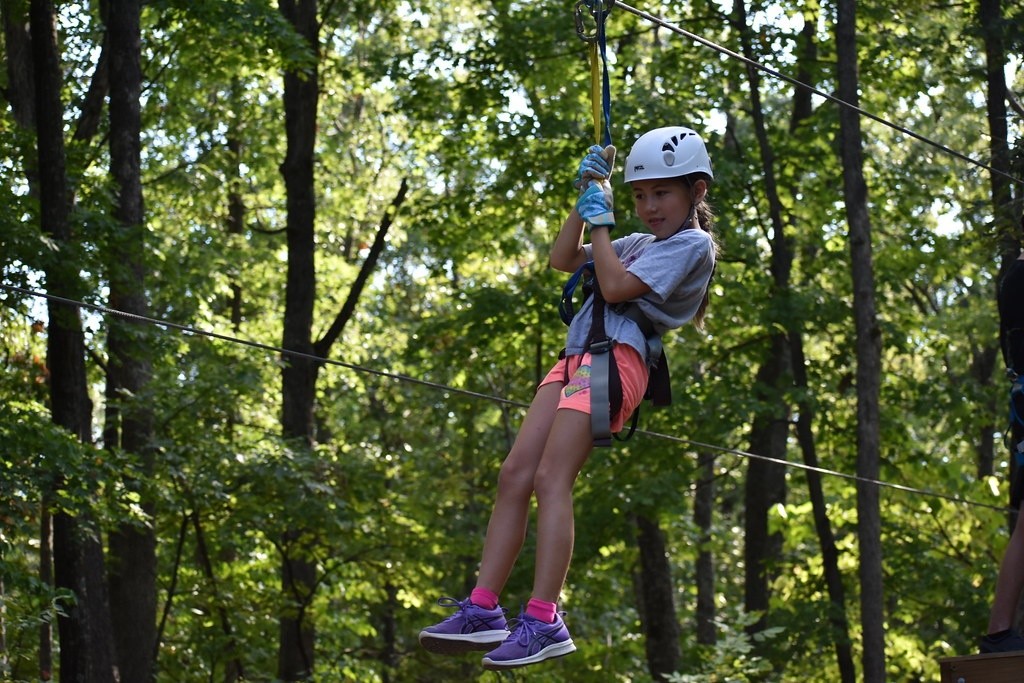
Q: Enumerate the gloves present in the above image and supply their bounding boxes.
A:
[574,144,616,192]
[575,179,616,233]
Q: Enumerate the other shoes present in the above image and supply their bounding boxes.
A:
[978,629,1024,654]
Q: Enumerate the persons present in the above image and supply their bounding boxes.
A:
[418,126,721,669]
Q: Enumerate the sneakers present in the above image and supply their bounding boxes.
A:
[419,597,512,655]
[481,603,577,671]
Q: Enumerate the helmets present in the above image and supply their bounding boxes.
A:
[624,126,714,184]
